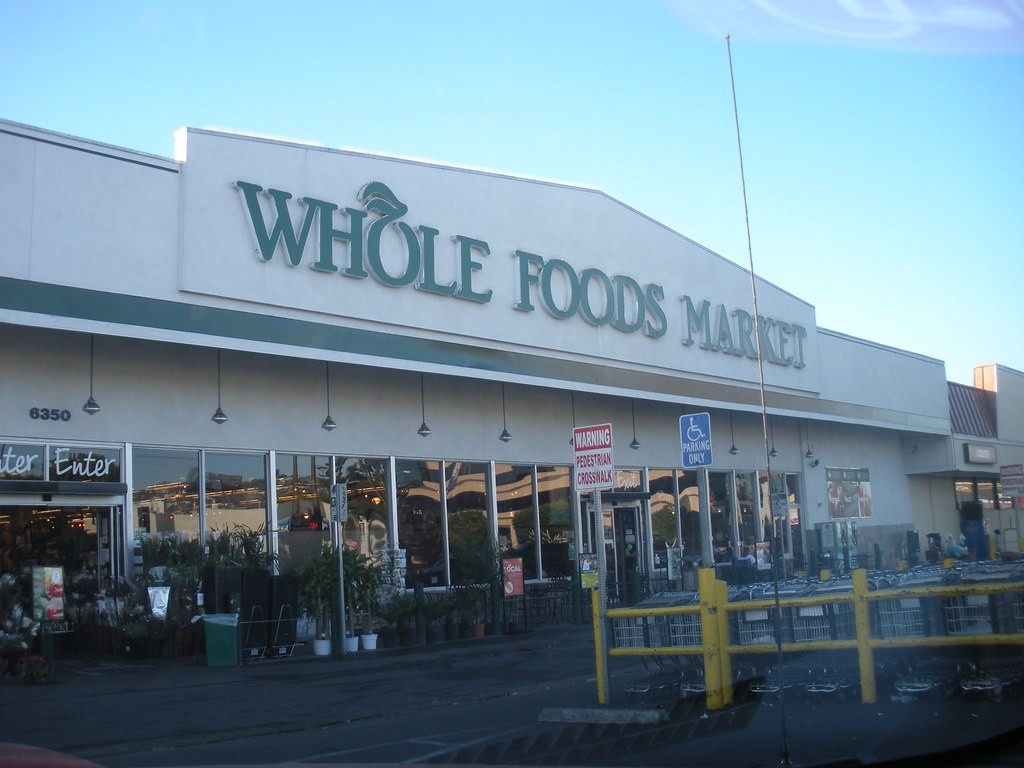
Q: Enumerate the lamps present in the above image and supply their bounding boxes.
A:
[805,418,813,458]
[729,410,738,455]
[211,348,228,424]
[630,398,640,450]
[499,381,512,442]
[82,335,100,415]
[417,372,431,437]
[769,414,778,458]
[321,361,337,431]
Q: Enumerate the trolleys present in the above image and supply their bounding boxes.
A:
[614,555,1023,706]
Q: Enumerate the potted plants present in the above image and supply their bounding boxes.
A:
[104,529,204,661]
[285,544,380,655]
[376,599,401,648]
[444,588,486,640]
[424,593,448,643]
[390,592,417,646]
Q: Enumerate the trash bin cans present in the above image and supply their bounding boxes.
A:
[733,559,757,585]
[204,613,239,666]
